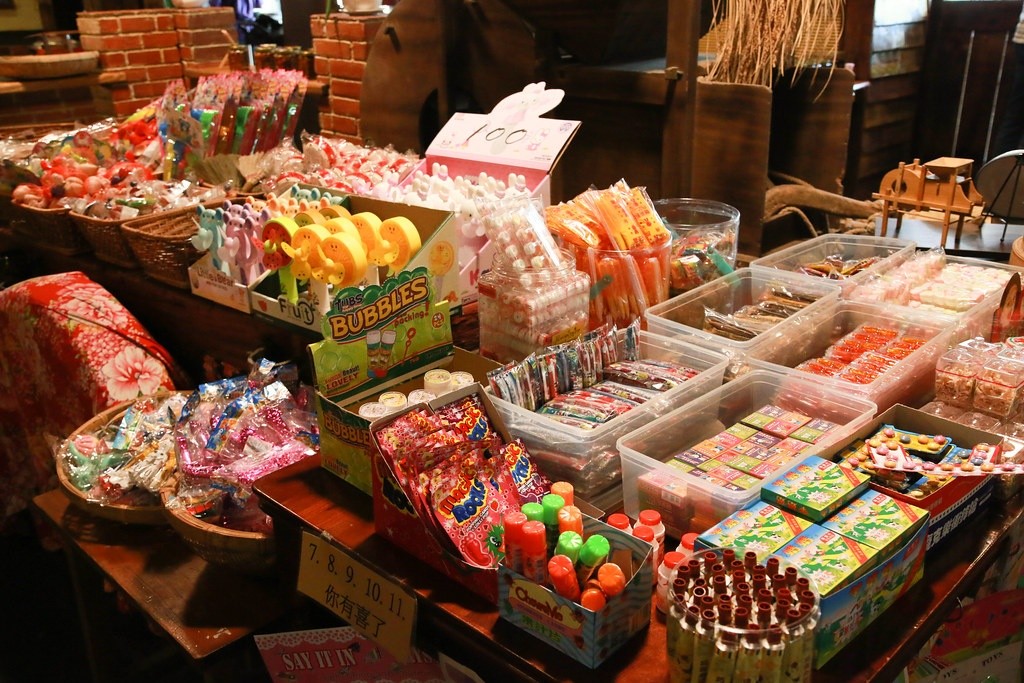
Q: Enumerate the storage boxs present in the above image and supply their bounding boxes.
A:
[183,78,1024,681]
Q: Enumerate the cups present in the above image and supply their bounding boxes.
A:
[566,229,674,332]
[654,197,739,295]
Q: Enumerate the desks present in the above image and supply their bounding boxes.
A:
[0,229,323,386]
[873,217,1024,262]
[32,485,298,683]
[252,214,1024,683]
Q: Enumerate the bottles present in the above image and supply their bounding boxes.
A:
[479,252,591,366]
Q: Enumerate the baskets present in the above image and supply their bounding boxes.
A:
[10,177,262,289]
